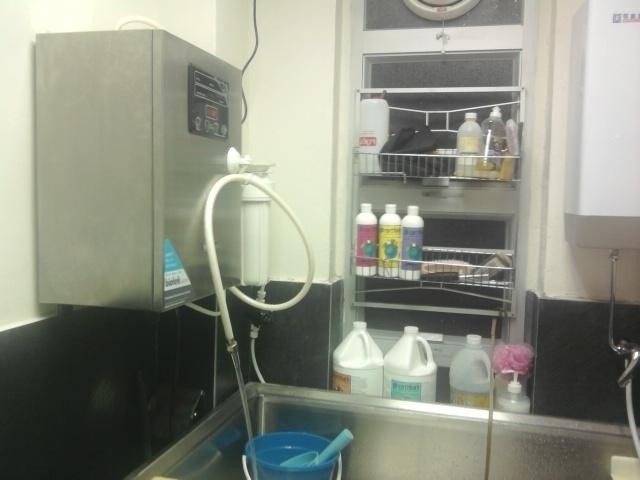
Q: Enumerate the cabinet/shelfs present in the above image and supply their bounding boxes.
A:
[351,83,521,320]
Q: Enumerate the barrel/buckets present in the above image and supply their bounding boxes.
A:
[449,333,495,409]
[242,431,342,480]
[330,320,383,398]
[383,325,438,402]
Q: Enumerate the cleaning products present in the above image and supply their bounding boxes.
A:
[403,205,424,280]
[350,204,381,276]
[380,205,403,279]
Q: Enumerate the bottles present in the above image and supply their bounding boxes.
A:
[353,202,424,281]
[453,111,486,177]
